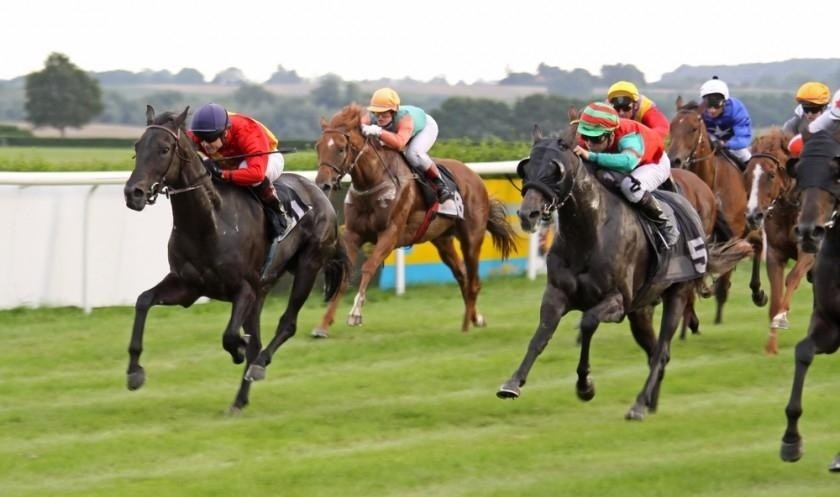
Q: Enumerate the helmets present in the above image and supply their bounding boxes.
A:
[366,88,400,114]
[575,101,620,137]
[606,81,641,102]
[795,82,832,106]
[189,102,230,134]
[699,80,731,101]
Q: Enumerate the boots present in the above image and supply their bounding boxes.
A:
[636,189,680,252]
[253,177,287,237]
[425,160,456,204]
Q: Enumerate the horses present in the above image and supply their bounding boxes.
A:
[309,102,528,339]
[124,104,355,417]
[495,95,840,471]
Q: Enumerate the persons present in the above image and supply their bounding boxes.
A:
[573,104,683,255]
[788,87,840,158]
[359,87,451,206]
[603,81,680,194]
[183,102,288,239]
[782,81,831,139]
[696,76,755,174]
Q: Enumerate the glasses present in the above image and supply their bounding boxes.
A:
[613,105,634,112]
[803,106,823,114]
[198,133,220,144]
[374,112,393,119]
[706,101,723,109]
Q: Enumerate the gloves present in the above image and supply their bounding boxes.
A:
[360,123,383,138]
[203,157,223,178]
